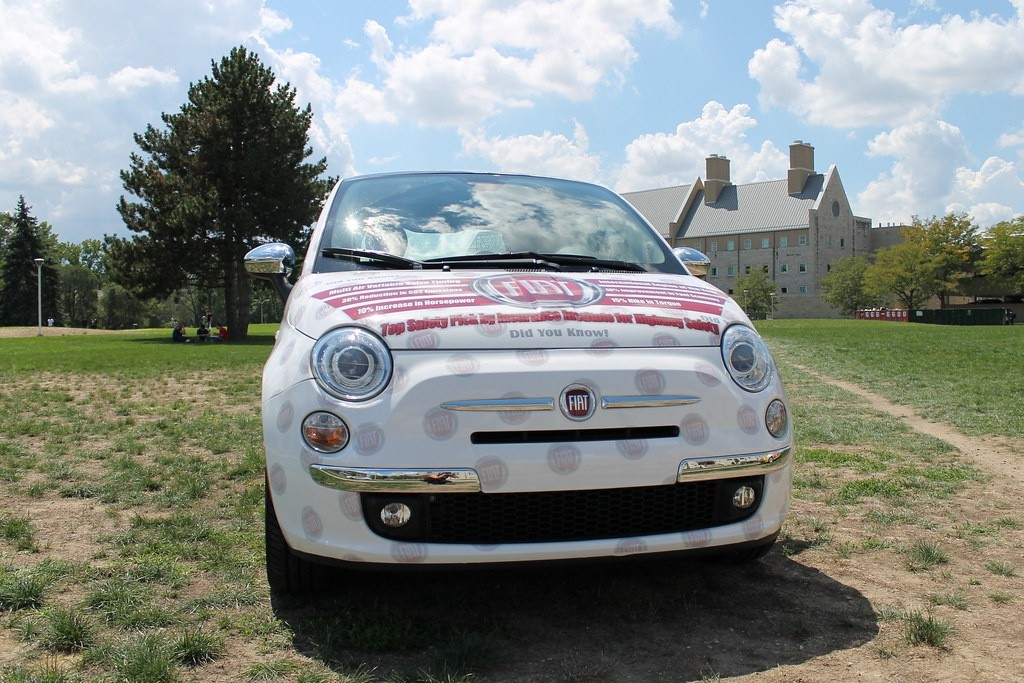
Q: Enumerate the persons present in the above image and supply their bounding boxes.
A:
[202,309,212,331]
[194,325,209,343]
[47,315,54,326]
[173,323,191,343]
[210,323,229,342]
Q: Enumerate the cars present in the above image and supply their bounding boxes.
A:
[243,168,794,598]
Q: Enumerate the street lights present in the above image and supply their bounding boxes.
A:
[743,290,748,313]
[770,292,776,320]
[35,257,45,338]
[254,299,270,324]
